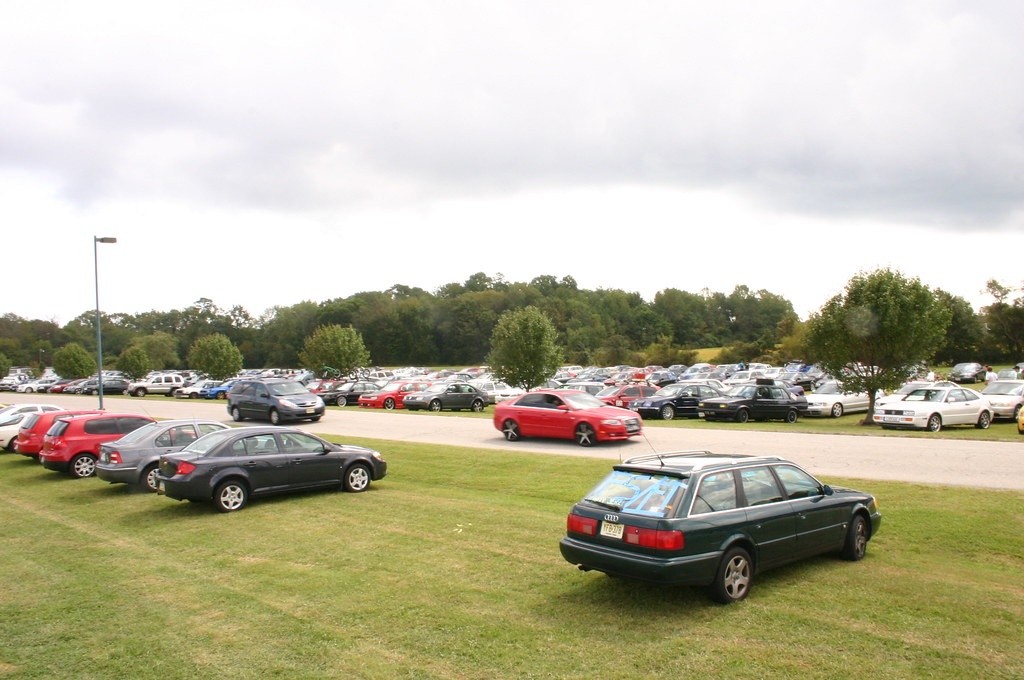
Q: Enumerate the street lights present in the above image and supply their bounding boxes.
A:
[92,234,117,410]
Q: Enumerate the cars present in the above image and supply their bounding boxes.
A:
[996,362,1024,380]
[95,419,280,494]
[1017,405,1024,434]
[873,380,962,414]
[627,383,726,421]
[36,414,199,479]
[696,383,810,424]
[872,386,995,433]
[402,382,492,413]
[946,362,987,384]
[0,360,930,411]
[153,426,389,515]
[0,403,66,452]
[978,379,1024,421]
[225,377,326,426]
[12,408,110,461]
[802,380,885,418]
[555,430,884,606]
[492,388,645,448]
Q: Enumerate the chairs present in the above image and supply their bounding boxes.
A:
[264,440,275,450]
[246,437,258,449]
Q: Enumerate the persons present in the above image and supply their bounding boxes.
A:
[985,366,998,385]
[926,371,933,382]
[738,359,749,371]
[1013,366,1024,379]
[799,364,807,372]
